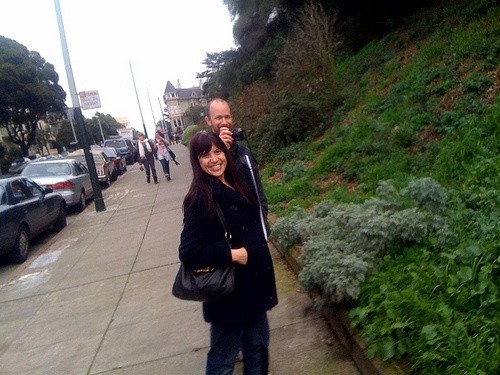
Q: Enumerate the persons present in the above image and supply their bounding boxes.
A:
[137,133,159,184]
[169,131,180,145]
[154,130,180,181]
[205,98,272,361]
[172,130,279,375]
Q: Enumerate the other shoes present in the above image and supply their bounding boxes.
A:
[166,177,171,181]
[176,162,180,165]
[165,174,168,177]
[147,176,159,184]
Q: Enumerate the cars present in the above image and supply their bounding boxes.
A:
[66,149,118,188]
[19,159,94,210]
[0,175,67,263]
[8,138,101,177]
[92,148,127,175]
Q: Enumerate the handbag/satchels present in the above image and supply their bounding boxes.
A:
[172,187,237,301]
[139,166,144,171]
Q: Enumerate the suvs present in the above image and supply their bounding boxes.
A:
[103,138,138,164]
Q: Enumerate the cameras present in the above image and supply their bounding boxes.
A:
[229,128,247,141]
